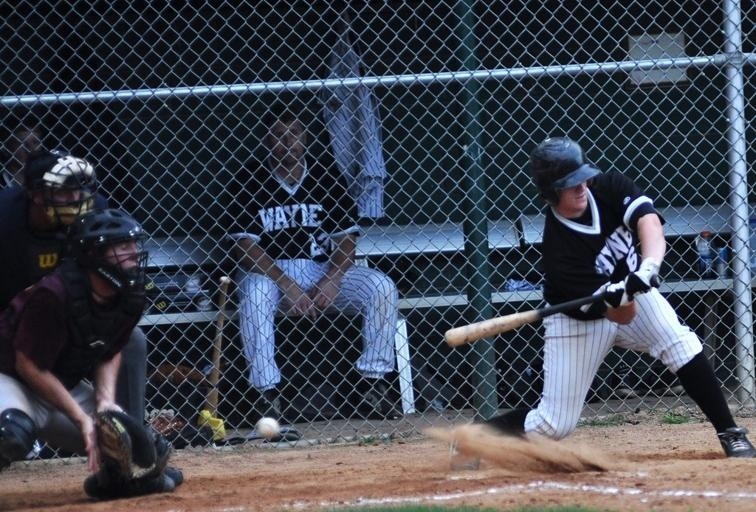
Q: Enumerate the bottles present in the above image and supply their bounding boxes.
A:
[504,278,540,292]
[144,409,181,432]
[696,231,715,278]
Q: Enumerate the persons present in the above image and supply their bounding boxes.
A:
[216,110,407,440]
[479,137,755,456]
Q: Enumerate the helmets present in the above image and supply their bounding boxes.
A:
[24,148,97,231]
[71,209,149,289]
[532,135,603,192]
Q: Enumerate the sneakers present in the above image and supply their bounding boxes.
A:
[448,427,480,471]
[355,375,404,420]
[255,390,282,423]
[716,426,756,458]
[83,467,184,499]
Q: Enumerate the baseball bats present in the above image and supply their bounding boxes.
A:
[444,275,660,347]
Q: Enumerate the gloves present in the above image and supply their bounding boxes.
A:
[601,259,660,307]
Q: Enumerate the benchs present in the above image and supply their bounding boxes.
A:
[125,199,756,417]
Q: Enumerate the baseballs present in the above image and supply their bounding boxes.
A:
[257,417,280,438]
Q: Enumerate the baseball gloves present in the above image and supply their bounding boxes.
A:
[95,409,170,492]
